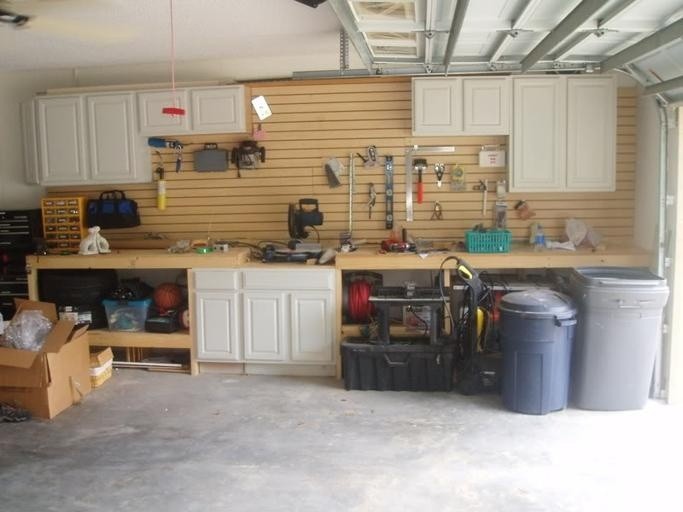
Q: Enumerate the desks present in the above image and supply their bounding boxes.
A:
[336,242,653,380]
[25,244,251,376]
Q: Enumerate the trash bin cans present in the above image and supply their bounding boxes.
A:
[497,267,669,415]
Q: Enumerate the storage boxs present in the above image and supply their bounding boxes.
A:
[0,298,114,421]
[340,335,458,392]
[99,297,153,333]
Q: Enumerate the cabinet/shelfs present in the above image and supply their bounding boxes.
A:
[18,82,249,186]
[412,74,618,194]
[242,268,336,379]
[192,270,242,374]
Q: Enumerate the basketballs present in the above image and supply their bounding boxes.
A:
[155,283,179,309]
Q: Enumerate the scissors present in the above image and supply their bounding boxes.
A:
[434,163,445,188]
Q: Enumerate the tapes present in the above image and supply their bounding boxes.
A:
[451,165,464,182]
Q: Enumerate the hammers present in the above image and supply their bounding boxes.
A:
[412,158,428,204]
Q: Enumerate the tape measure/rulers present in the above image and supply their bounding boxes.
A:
[405,147,455,222]
